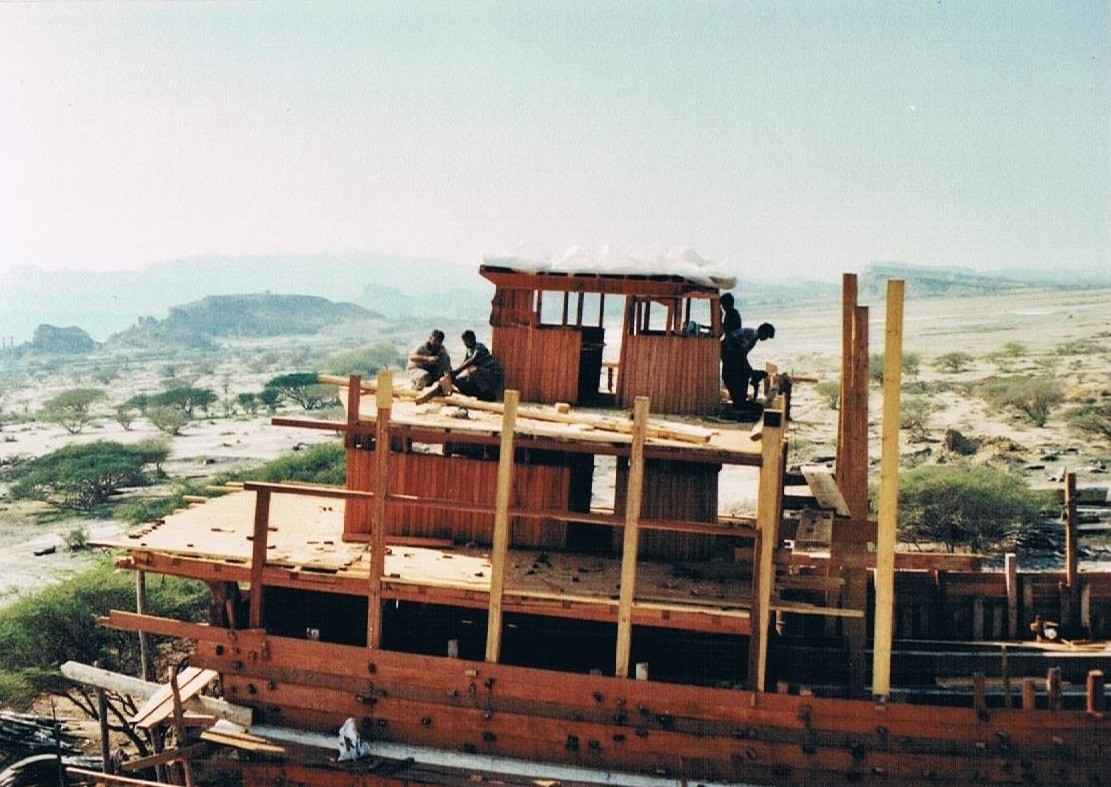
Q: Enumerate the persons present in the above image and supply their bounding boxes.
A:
[721,322,775,415]
[448,330,504,401]
[720,293,742,332]
[409,330,456,396]
